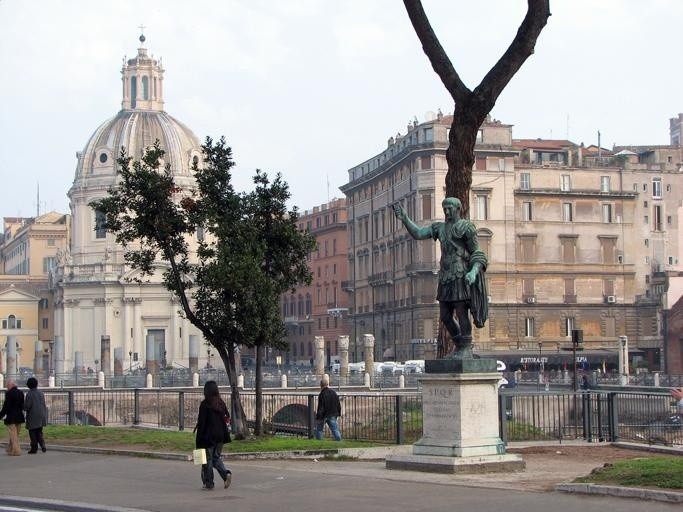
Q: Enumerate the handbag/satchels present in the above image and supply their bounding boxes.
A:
[191,448,207,465]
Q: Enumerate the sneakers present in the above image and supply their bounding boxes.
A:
[5,448,46,456]
[222,469,232,489]
[202,483,215,489]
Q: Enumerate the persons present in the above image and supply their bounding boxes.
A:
[668,387,682,416]
[579,374,591,389]
[87,368,94,375]
[20,377,47,454]
[194,380,232,490]
[80,366,86,376]
[391,196,489,360]
[313,378,342,440]
[0,380,24,456]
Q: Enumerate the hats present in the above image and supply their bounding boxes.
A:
[6,379,17,390]
